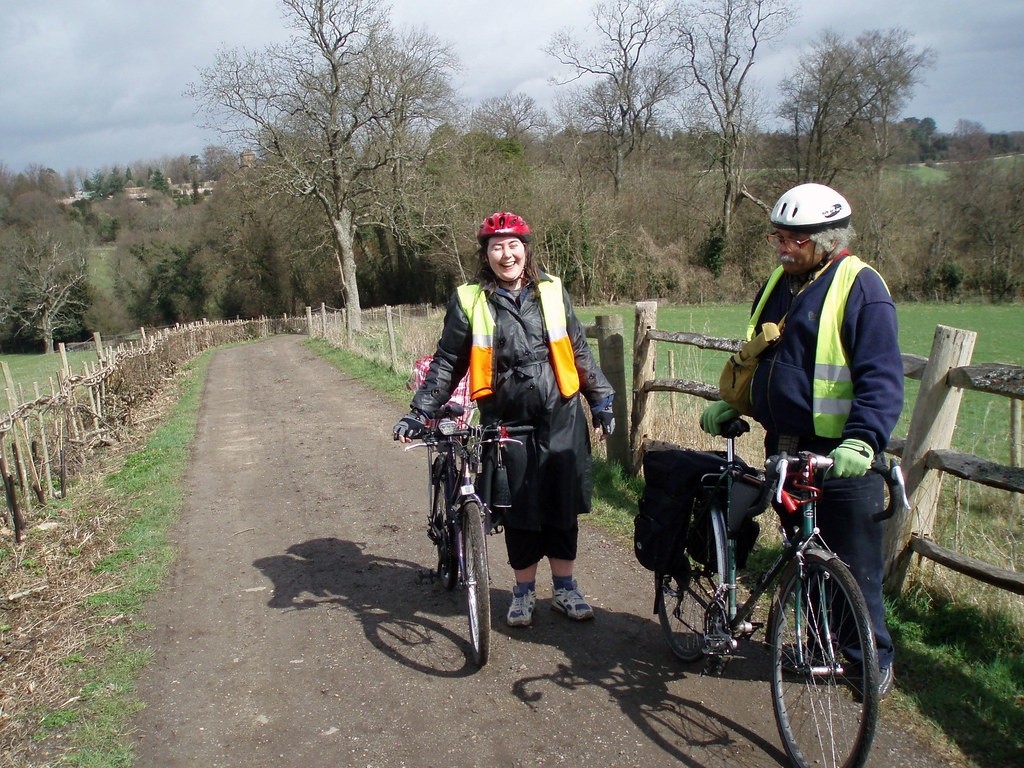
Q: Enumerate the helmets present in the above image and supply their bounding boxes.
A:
[770,183,852,232]
[477,213,530,247]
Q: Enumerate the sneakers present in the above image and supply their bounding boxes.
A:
[852,659,893,703]
[551,577,594,621]
[507,584,535,627]
[788,640,836,662]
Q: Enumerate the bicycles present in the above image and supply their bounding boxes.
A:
[407,400,538,670]
[654,412,912,768]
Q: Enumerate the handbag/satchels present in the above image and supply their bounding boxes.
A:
[633,447,757,575]
[719,324,782,417]
[407,354,474,431]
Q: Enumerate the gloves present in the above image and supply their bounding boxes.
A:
[699,398,741,436]
[823,438,874,478]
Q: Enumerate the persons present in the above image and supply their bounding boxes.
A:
[700,183,904,701]
[393,210,615,627]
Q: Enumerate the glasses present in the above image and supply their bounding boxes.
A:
[766,231,810,252]
